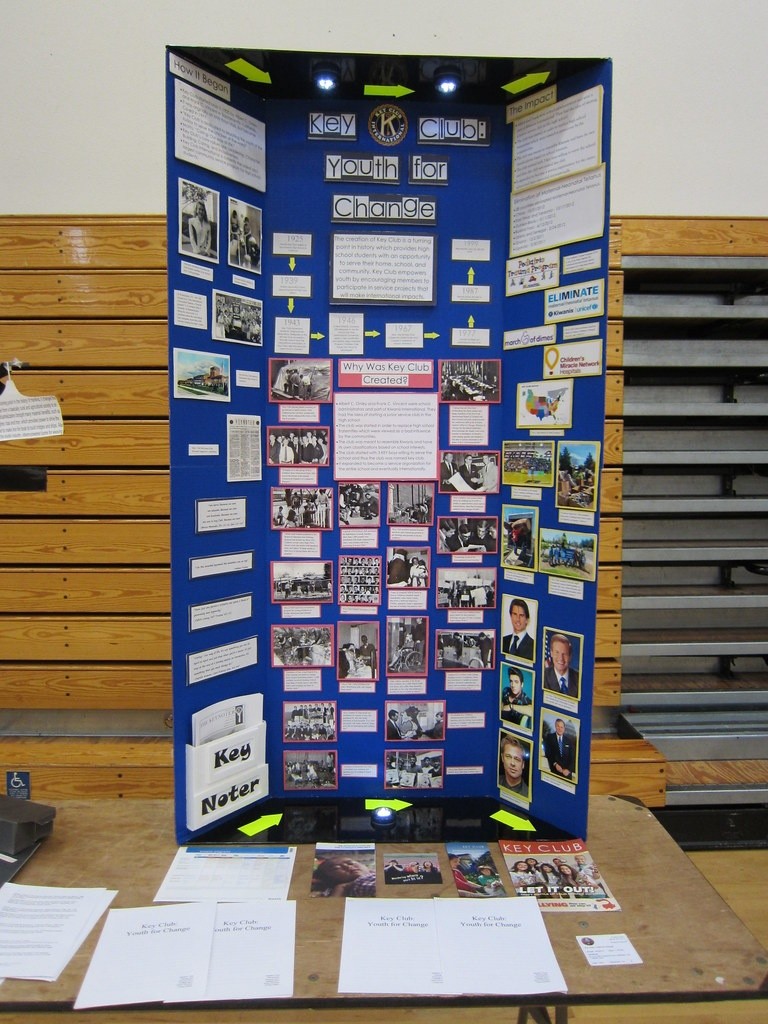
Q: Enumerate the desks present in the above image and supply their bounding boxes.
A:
[1,793,768,1024]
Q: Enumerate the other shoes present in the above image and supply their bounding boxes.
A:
[364,516,372,520]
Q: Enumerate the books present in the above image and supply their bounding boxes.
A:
[499,838,622,912]
[310,842,377,898]
[445,841,507,898]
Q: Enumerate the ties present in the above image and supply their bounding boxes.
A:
[509,636,519,654]
[449,463,451,474]
[561,677,567,694]
[486,466,487,472]
[558,737,562,753]
[468,465,469,472]
[285,446,287,461]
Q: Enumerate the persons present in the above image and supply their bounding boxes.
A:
[271,360,596,797]
[384,859,442,884]
[189,198,260,344]
[446,853,503,896]
[509,853,610,899]
[316,856,376,896]
